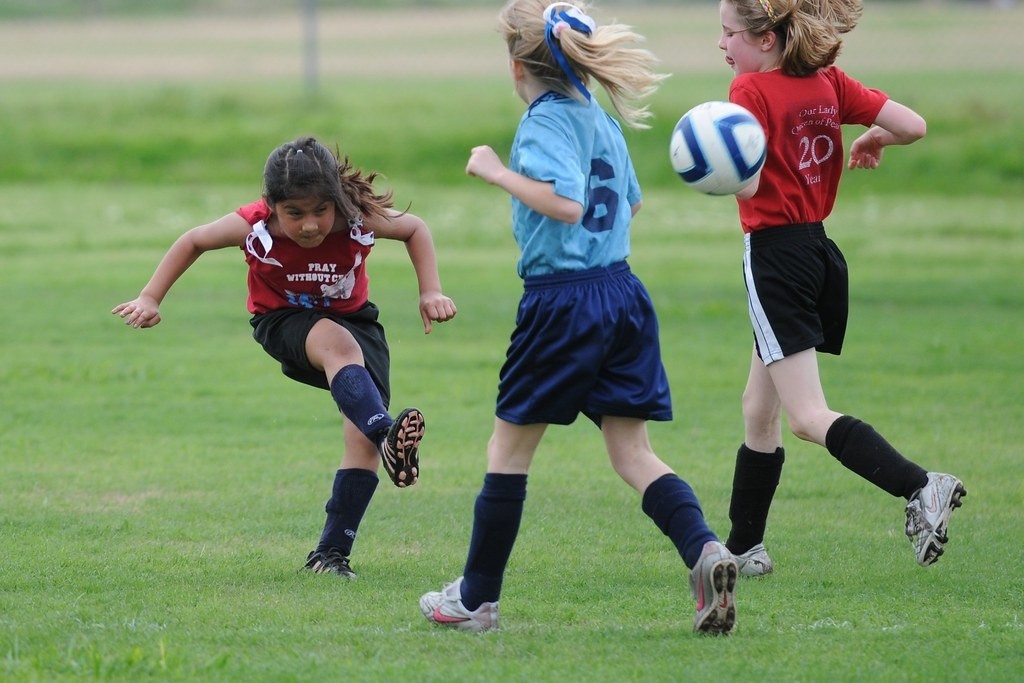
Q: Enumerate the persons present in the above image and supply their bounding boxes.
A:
[110,136,457,580]
[718,0,967,578]
[419,0,738,638]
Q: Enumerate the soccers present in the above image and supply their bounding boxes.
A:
[668,99,769,198]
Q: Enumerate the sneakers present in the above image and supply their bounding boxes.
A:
[305,551,356,581]
[722,544,774,579]
[690,541,738,638]
[904,471,967,568]
[380,408,426,488]
[419,576,501,635]
